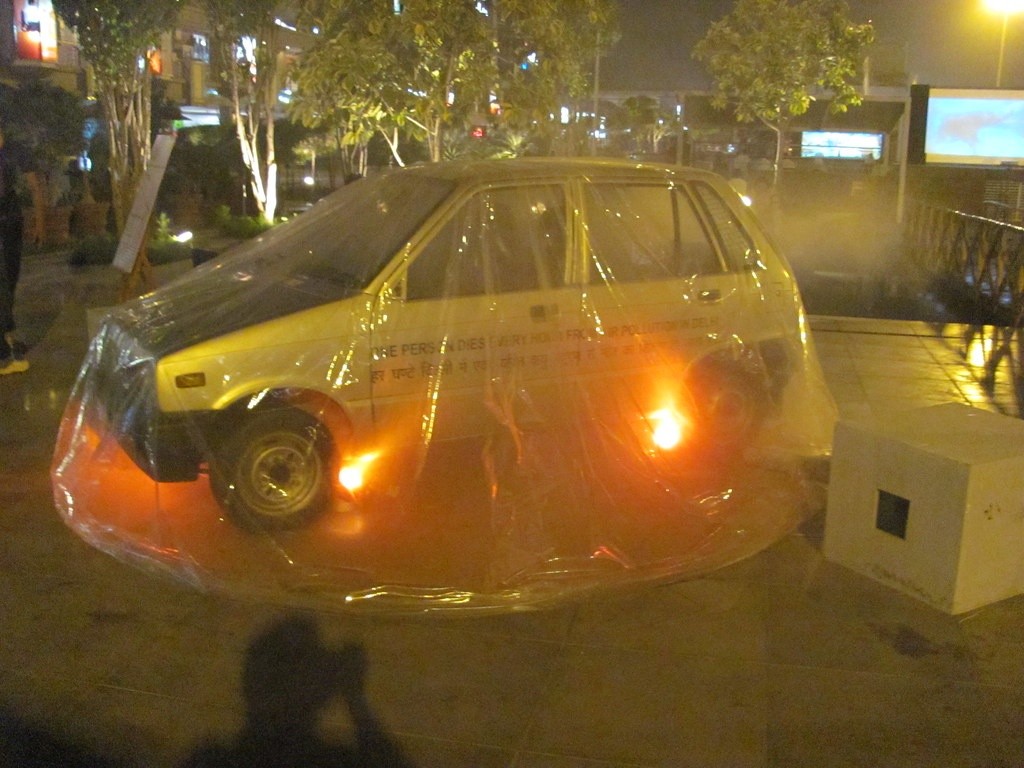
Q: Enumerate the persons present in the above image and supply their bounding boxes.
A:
[57,159,88,209]
[1,132,31,377]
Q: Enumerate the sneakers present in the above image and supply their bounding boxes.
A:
[0,358,30,375]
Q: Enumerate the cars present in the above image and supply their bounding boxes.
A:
[97,156,806,538]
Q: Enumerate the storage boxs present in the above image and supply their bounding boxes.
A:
[821,400,1023,615]
